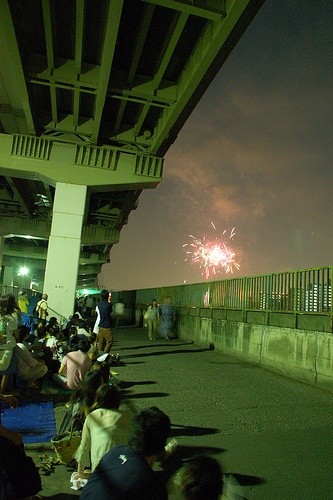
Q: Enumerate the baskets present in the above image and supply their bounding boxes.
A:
[50,418,82,465]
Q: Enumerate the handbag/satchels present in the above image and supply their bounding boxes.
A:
[144,306,151,320]
[35,300,45,316]
[54,395,86,434]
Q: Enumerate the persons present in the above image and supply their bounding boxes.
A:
[158,296,176,340]
[0,289,120,407]
[92,361,112,383]
[79,406,172,500]
[98,289,115,353]
[0,423,42,500]
[17,292,29,326]
[176,455,224,500]
[68,370,104,432]
[35,294,49,340]
[144,299,160,340]
[73,385,177,479]
[13,326,65,386]
[54,339,92,394]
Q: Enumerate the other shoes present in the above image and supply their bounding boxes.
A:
[166,337,169,341]
[153,337,156,341]
[149,337,152,341]
